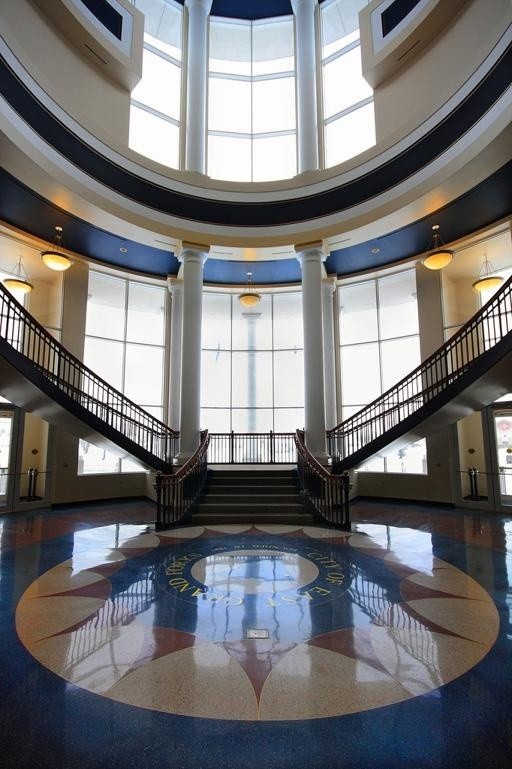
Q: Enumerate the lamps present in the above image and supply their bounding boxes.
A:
[471,253,505,292]
[2,256,34,293]
[40,223,74,273]
[420,224,454,271]
[239,271,261,306]
